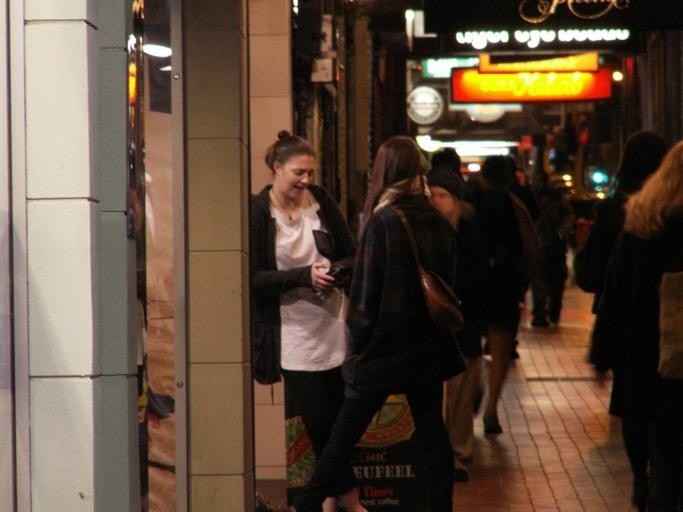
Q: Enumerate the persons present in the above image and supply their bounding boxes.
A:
[512,168,528,309]
[570,130,668,512]
[467,156,530,436]
[591,138,682,512]
[284,135,467,511]
[425,167,472,483]
[529,171,567,333]
[482,155,541,360]
[249,124,370,512]
[430,148,464,182]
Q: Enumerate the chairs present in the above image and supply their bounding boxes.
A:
[483,409,501,432]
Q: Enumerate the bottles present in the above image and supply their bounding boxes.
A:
[425,165,466,201]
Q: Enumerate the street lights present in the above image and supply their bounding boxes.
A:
[416,264,466,332]
